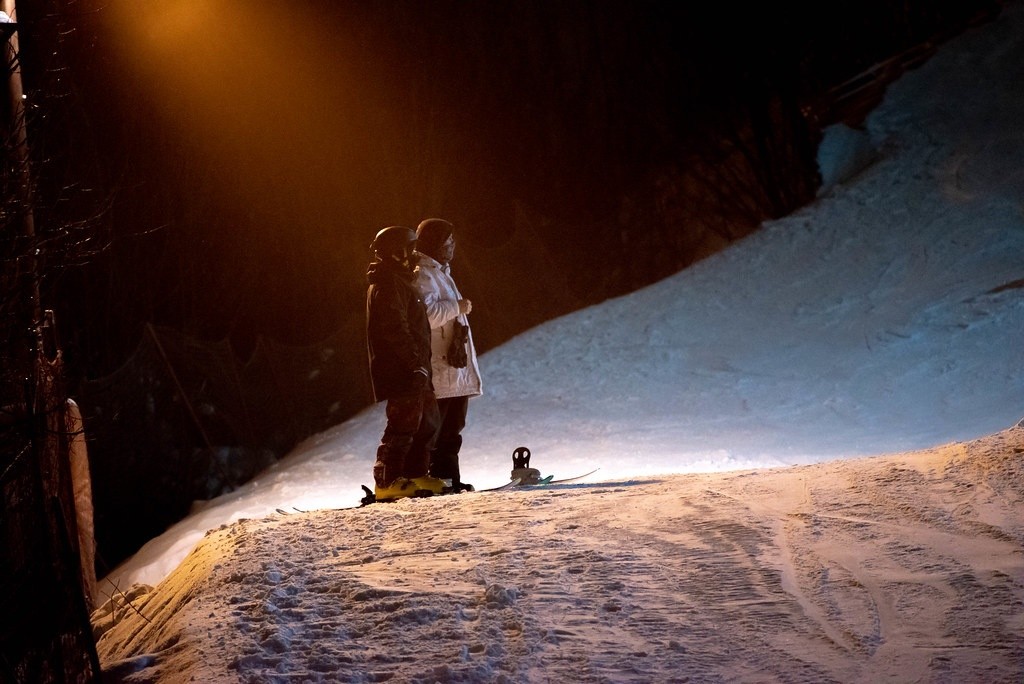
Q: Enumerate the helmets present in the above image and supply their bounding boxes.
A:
[374,227,419,253]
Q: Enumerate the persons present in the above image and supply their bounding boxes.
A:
[366,219,482,498]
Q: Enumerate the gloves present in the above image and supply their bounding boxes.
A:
[447,316,469,369]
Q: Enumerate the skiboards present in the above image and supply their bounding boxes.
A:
[277,473,554,514]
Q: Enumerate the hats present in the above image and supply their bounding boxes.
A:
[416,219,454,248]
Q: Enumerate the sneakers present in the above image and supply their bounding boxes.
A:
[375,477,449,503]
[449,479,475,494]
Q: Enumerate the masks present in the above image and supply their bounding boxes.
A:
[406,254,421,271]
[433,242,455,264]
